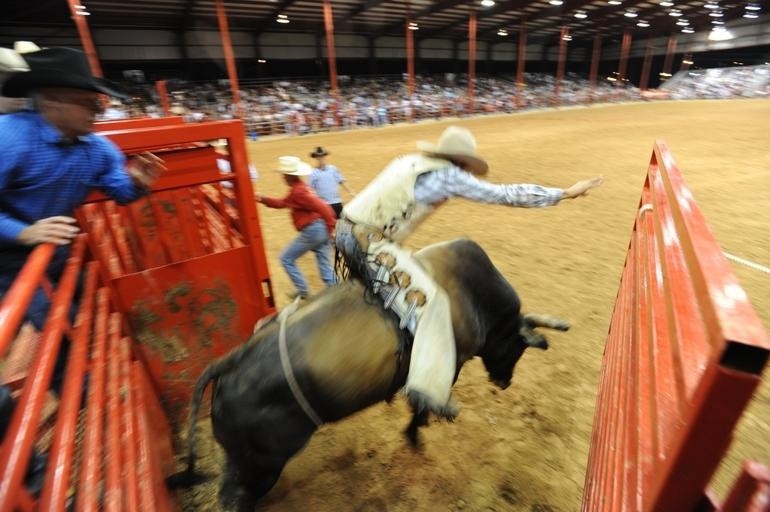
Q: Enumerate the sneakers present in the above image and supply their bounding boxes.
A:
[285,291,311,301]
[408,389,465,420]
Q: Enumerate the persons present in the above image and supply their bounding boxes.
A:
[210,139,259,190]
[96,60,619,134]
[0,46,167,408]
[306,146,355,218]
[659,65,770,100]
[334,125,605,449]
[0,386,105,512]
[252,156,341,300]
[0,47,31,112]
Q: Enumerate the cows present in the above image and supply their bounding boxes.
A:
[163,235,572,512]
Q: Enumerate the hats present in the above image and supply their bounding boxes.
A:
[309,146,328,158]
[0,43,137,101]
[415,124,489,177]
[267,154,314,177]
